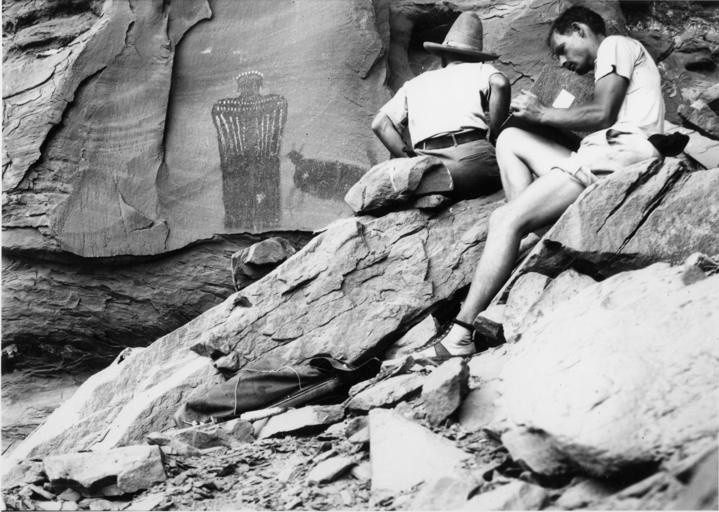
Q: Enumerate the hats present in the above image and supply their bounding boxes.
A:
[423,11,500,60]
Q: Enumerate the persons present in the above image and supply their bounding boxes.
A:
[408,5,667,362]
[372,13,516,200]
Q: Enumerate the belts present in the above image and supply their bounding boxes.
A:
[415,128,486,150]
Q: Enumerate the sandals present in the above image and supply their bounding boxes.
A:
[401,320,477,363]
